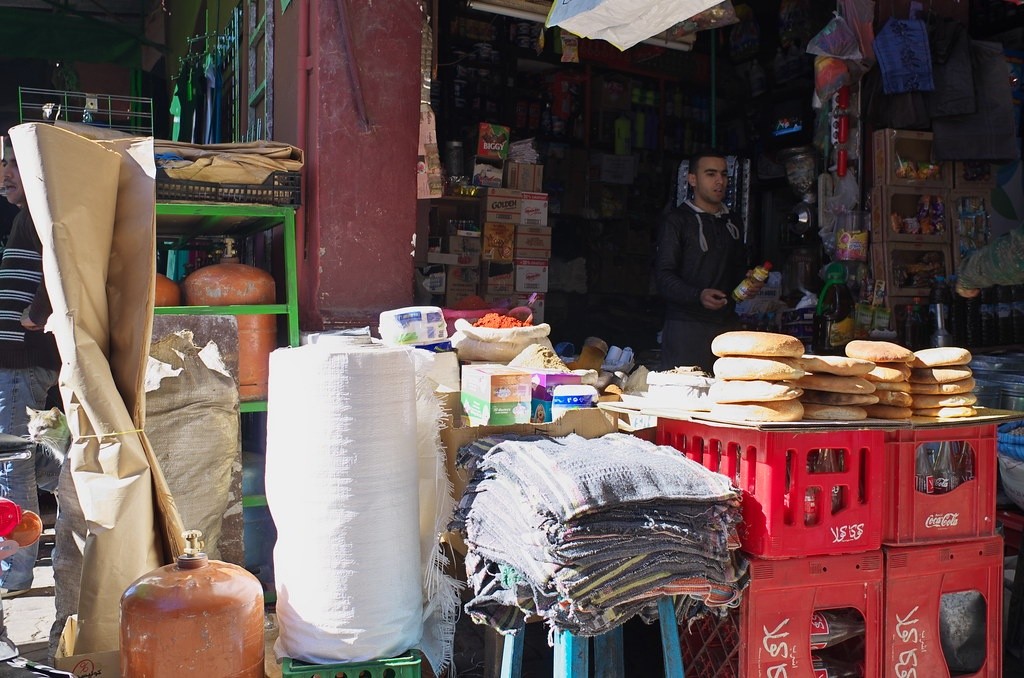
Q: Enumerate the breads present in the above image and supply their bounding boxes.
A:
[708,330,977,422]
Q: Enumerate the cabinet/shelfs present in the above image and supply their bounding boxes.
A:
[153,202,301,609]
[435,0,716,320]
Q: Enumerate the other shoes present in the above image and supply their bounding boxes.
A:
[0,583,32,598]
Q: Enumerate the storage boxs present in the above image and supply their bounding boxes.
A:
[434,119,1024,678]
[279,648,423,678]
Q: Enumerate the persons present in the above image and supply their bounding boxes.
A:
[655,148,762,373]
[0,137,62,598]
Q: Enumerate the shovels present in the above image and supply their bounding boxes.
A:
[506,291,540,323]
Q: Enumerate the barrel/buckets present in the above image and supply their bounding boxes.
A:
[967,354,1024,410]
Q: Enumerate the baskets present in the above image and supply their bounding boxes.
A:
[156,156,302,208]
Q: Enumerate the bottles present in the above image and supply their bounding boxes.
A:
[669,436,843,526]
[915,441,974,495]
[446,141,463,176]
[576,337,607,374]
[811,272,852,357]
[929,275,1024,346]
[932,303,950,347]
[731,261,772,304]
[902,298,925,347]
[810,609,867,678]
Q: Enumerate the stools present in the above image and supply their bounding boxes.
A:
[499,593,686,678]
